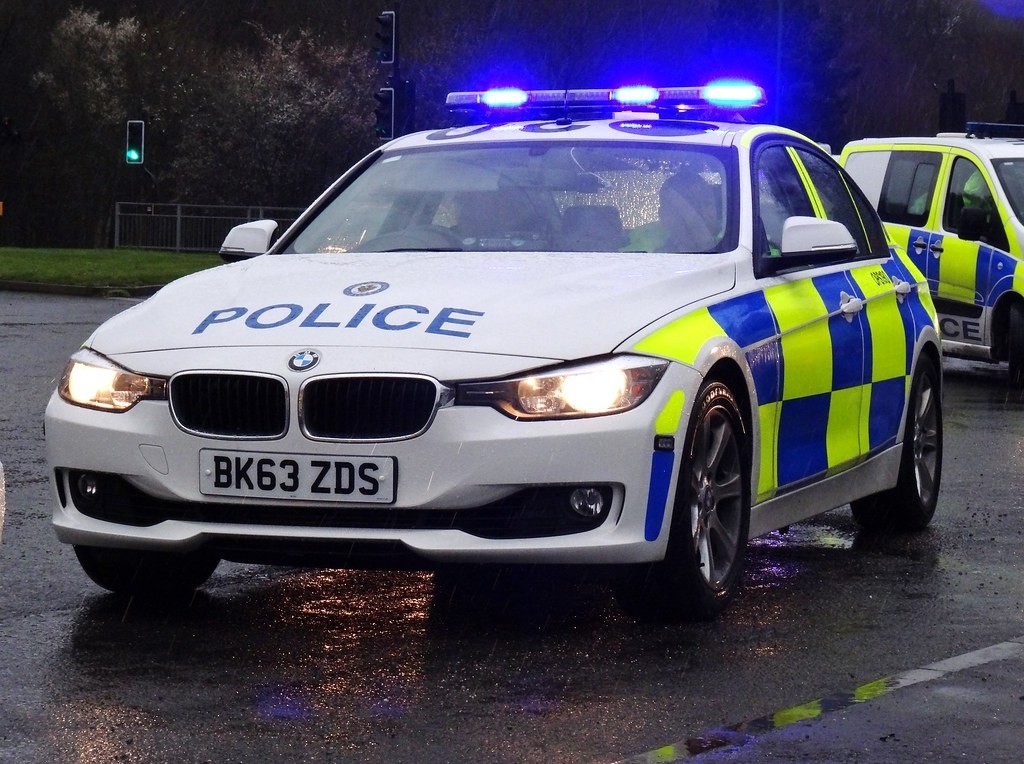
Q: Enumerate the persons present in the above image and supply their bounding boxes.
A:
[621,172,779,256]
[908,169,993,223]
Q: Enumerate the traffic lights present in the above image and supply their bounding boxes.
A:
[374,90,395,142]
[374,10,398,66]
[126,121,145,165]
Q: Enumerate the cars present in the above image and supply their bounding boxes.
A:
[40,82,948,627]
[838,121,1024,386]
